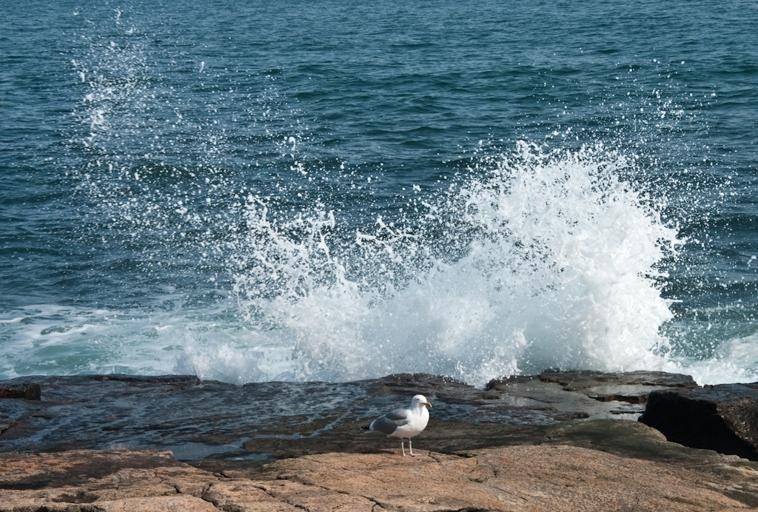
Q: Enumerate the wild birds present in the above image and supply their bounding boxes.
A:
[361,393,433,457]
